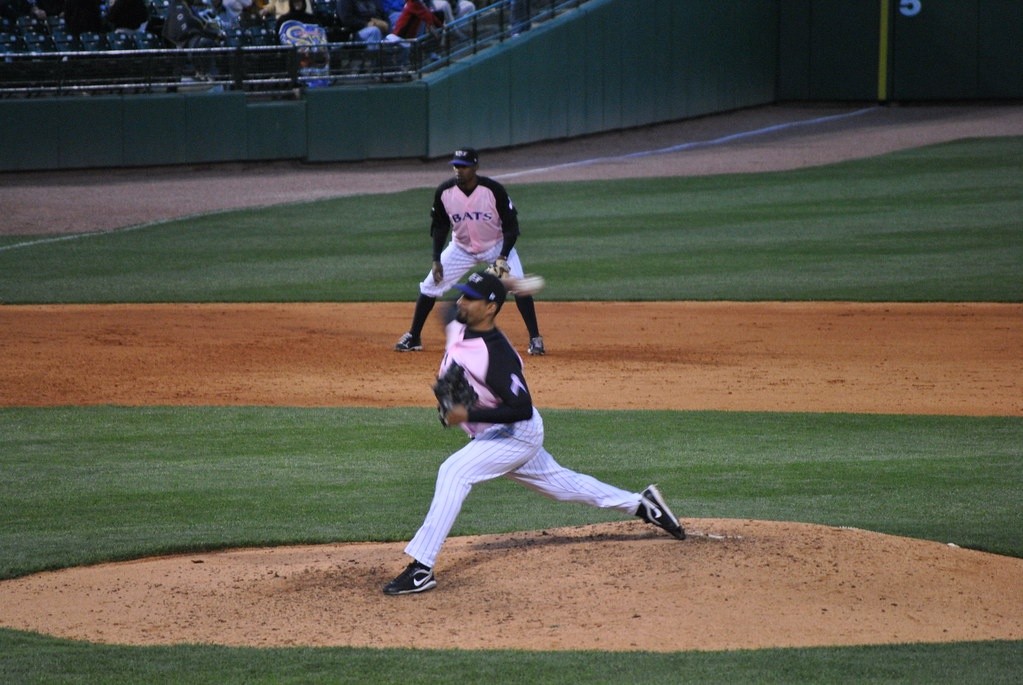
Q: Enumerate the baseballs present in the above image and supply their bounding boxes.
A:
[515,275,545,292]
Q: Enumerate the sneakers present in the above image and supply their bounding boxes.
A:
[641,484,686,539]
[528,336,545,355]
[394,332,422,351]
[381,559,437,594]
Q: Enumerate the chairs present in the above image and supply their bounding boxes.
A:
[0,0,355,63]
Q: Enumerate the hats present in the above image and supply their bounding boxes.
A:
[450,147,478,166]
[452,273,506,305]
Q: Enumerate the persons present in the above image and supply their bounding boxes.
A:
[391,145,547,359]
[382,262,686,597]
[0,0,476,92]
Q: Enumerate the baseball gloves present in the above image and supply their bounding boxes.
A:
[482,259,511,280]
[433,364,478,429]
